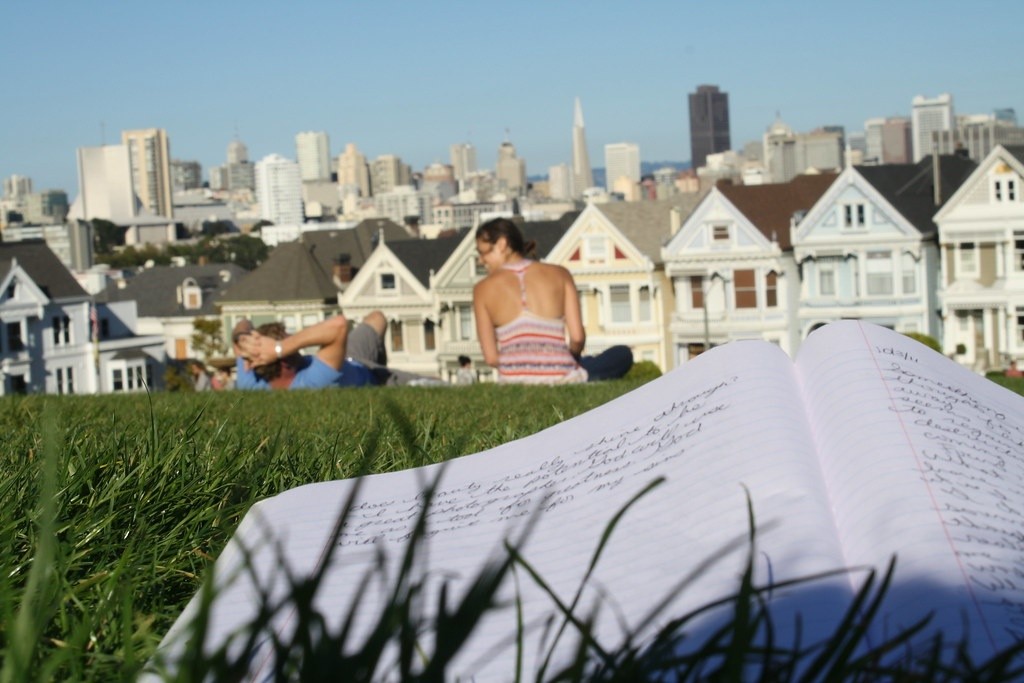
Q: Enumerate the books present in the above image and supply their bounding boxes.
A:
[138,318,1024,683]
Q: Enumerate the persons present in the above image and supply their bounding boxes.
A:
[189,360,213,392]
[472,216,590,386]
[453,355,475,386]
[230,310,392,390]
[212,363,236,391]
[1004,360,1023,378]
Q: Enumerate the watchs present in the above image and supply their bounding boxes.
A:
[274,339,283,359]
